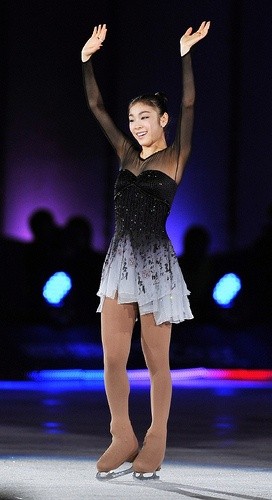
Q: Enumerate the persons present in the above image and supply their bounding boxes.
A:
[82,20,211,474]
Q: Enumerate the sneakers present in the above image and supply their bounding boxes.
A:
[96,432,167,481]
